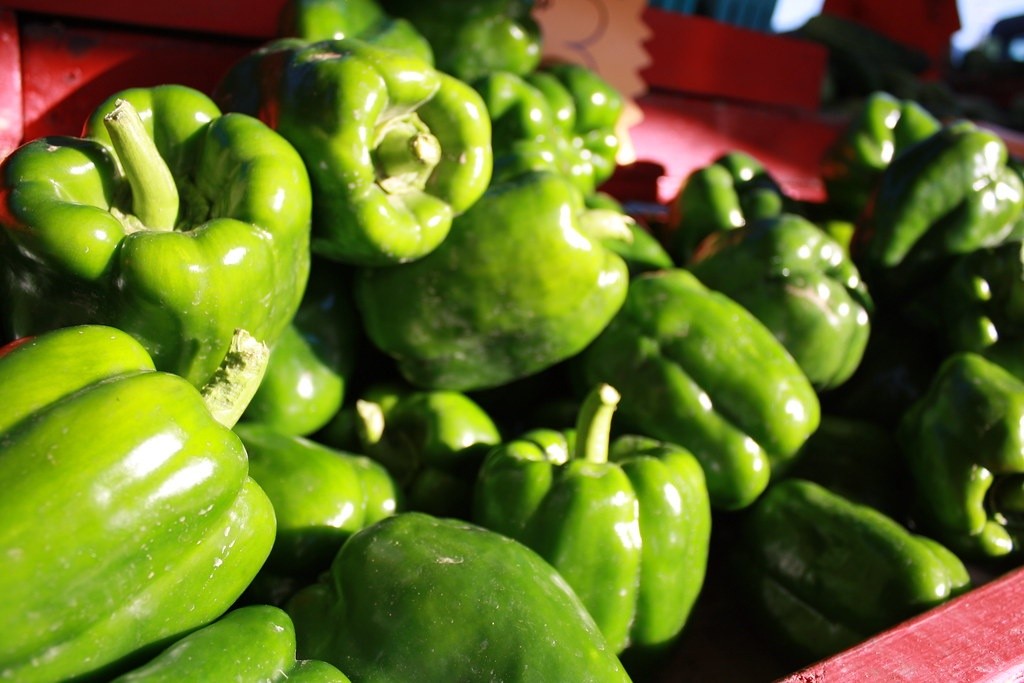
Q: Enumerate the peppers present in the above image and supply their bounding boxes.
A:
[0,0,1024,683]
